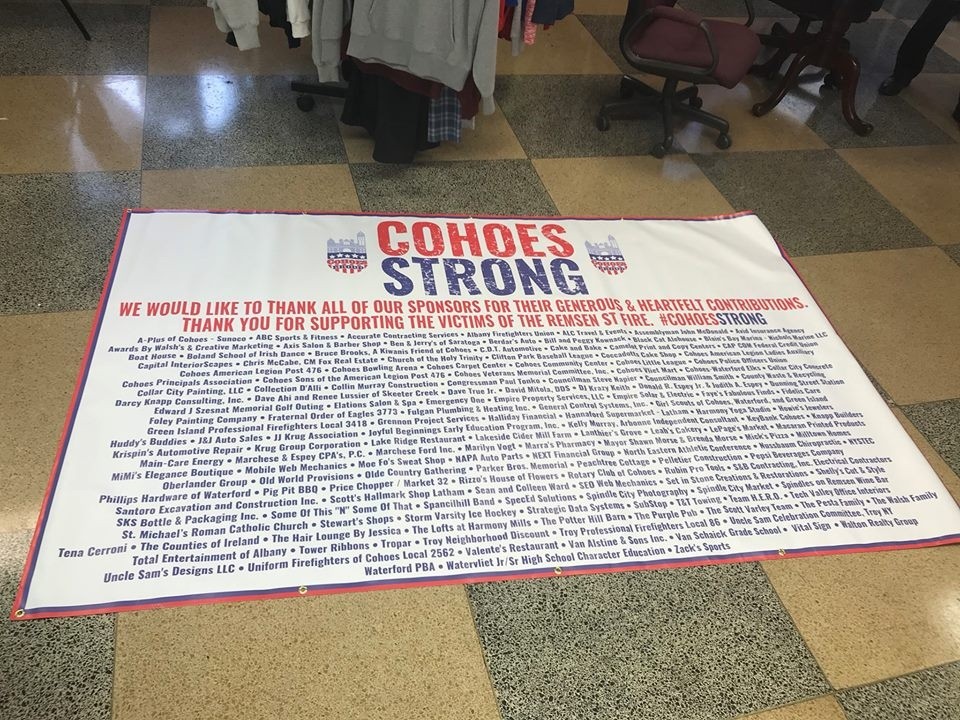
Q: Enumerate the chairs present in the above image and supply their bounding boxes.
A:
[593,0,758,159]
[748,0,884,138]
